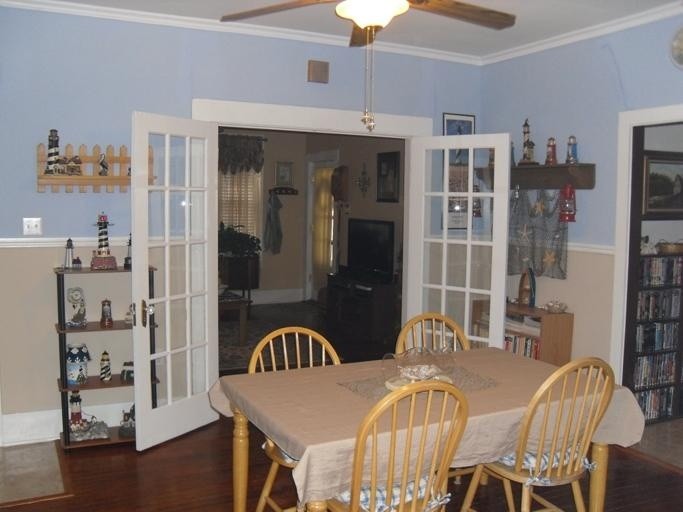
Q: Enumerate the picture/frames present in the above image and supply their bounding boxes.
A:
[642,149,683,220]
[442,112,476,231]
[274,160,294,187]
[376,150,400,203]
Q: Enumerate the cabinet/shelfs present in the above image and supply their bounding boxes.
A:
[471,298,574,367]
[325,272,396,345]
[632,254,683,427]
[53,265,157,452]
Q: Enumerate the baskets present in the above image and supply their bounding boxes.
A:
[654,239,683,255]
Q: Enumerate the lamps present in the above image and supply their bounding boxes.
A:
[335,0,409,31]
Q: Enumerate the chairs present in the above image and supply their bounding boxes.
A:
[328,379,470,512]
[396,313,471,484]
[248,327,343,511]
[461,356,615,512]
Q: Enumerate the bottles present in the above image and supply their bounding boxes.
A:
[99,350,111,383]
[543,137,557,167]
[98,297,112,329]
[62,239,74,268]
[40,129,63,175]
[565,135,578,165]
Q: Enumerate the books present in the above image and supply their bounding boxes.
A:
[633,254,682,422]
[471,310,541,360]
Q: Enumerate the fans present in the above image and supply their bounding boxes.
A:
[220,0,516,47]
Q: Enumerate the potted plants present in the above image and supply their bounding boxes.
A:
[219,221,262,289]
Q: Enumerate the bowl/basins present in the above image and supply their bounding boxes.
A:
[655,243,682,255]
[382,346,459,392]
[542,301,567,314]
[218,284,229,297]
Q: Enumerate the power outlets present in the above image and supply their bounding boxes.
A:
[22,218,41,236]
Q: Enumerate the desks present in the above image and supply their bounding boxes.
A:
[207,345,646,512]
[219,288,253,346]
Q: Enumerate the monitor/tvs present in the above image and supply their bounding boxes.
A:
[347,218,399,285]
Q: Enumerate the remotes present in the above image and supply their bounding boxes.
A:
[355,284,372,291]
